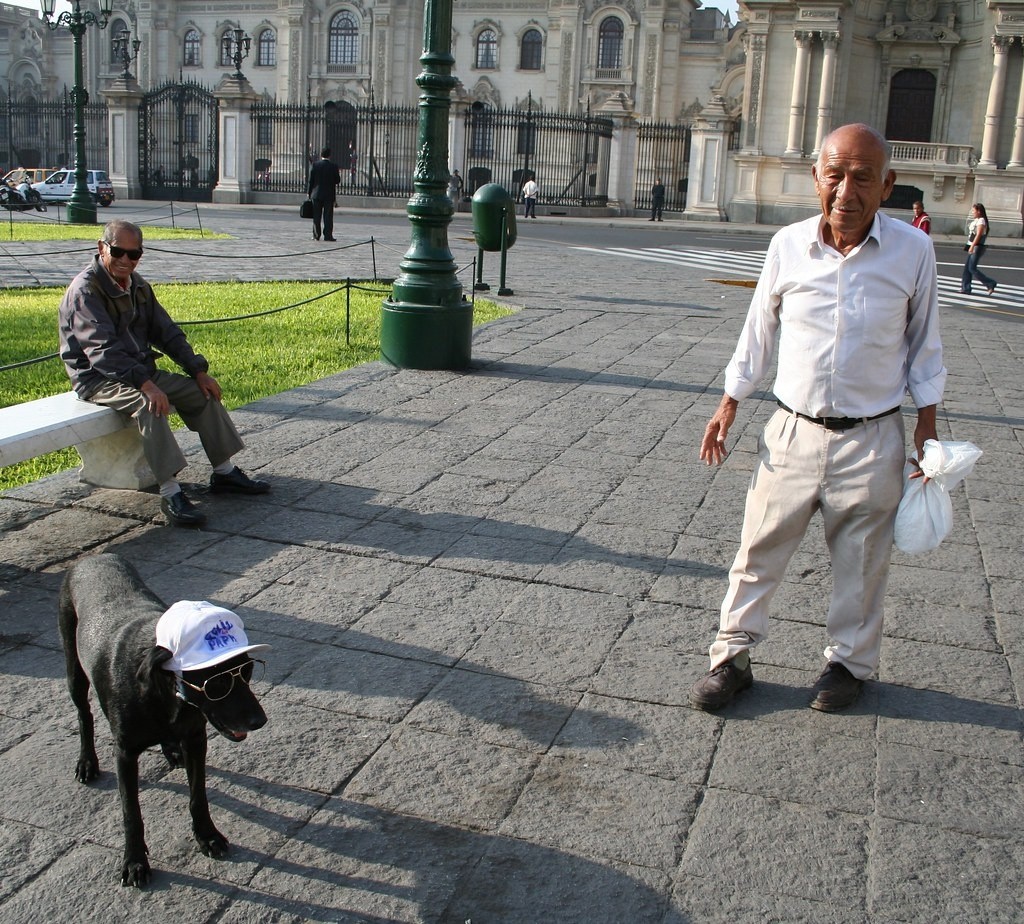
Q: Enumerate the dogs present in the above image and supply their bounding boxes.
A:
[57,551,272,890]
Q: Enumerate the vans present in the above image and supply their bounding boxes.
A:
[2,168,57,187]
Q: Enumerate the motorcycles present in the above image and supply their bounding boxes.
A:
[0,167,48,213]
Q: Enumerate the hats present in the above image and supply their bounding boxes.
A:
[656,177,661,181]
[153,600,272,671]
[453,169,459,173]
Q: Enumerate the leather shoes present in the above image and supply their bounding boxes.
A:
[160,491,207,525]
[208,466,271,494]
[689,655,755,710]
[807,660,865,713]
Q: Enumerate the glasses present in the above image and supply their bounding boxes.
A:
[105,242,143,260]
[174,657,267,702]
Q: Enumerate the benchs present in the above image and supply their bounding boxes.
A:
[0,391,177,489]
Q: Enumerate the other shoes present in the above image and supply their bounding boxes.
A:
[658,216,663,221]
[311,237,319,241]
[987,283,997,295]
[325,238,336,241]
[648,216,655,221]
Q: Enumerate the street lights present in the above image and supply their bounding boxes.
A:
[41,0,115,223]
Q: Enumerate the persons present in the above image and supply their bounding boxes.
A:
[912,201,931,235]
[956,203,997,297]
[58,219,272,522]
[306,148,342,241]
[523,175,540,219]
[650,177,665,221]
[692,124,947,714]
[449,170,464,210]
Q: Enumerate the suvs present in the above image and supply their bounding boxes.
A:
[32,169,115,206]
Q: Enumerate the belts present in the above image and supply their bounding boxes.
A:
[776,399,901,430]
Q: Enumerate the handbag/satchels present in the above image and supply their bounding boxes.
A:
[892,438,983,552]
[969,233,988,245]
[299,198,314,219]
